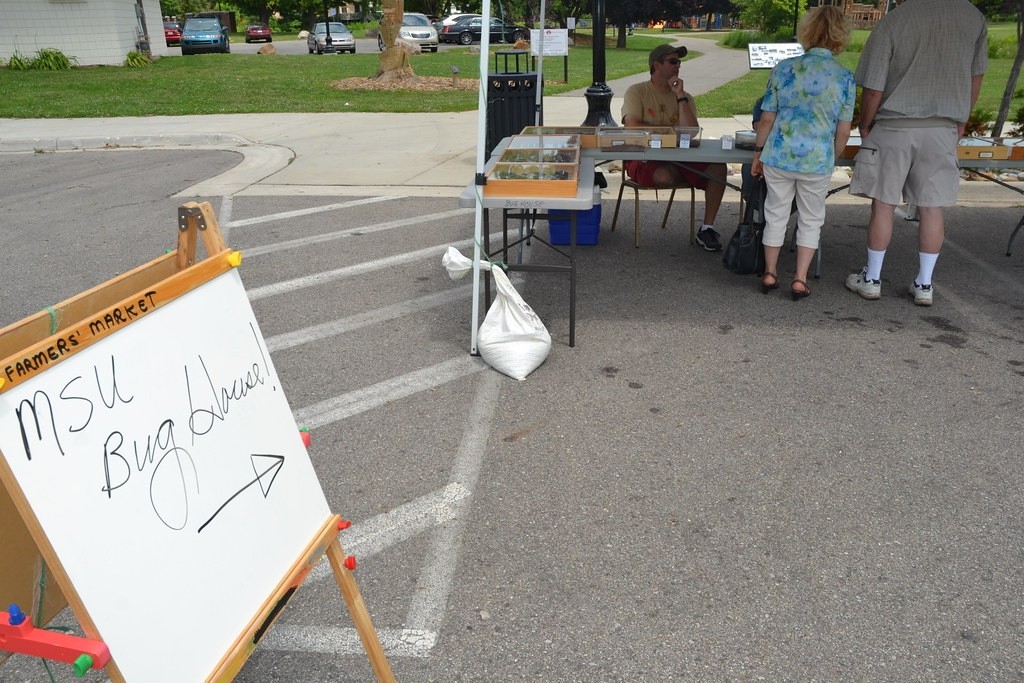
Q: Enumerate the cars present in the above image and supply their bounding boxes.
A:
[425,13,495,44]
[377,12,438,52]
[438,16,531,45]
[164,21,182,47]
[244,22,272,43]
[308,22,355,55]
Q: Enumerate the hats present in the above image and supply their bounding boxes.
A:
[649,44,687,67]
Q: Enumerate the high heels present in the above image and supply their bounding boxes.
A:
[761,272,781,292]
[791,280,810,302]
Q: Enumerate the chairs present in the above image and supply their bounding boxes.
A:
[611,125,696,247]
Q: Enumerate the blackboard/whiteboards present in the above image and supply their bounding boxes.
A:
[0,249,346,683]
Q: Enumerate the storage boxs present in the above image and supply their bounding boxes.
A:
[736,126,1024,160]
[483,125,707,248]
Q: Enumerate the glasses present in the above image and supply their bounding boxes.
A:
[665,57,681,66]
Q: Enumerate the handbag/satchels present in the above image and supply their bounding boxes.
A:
[723,174,768,276]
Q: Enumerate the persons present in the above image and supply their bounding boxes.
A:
[740,77,798,228]
[629,22,638,30]
[844,0,989,307]
[750,6,857,300]
[622,45,727,251]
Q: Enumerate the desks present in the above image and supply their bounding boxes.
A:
[492,138,768,247]
[484,155,597,347]
[791,136,1024,278]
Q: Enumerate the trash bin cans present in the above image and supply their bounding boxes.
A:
[485,50,544,163]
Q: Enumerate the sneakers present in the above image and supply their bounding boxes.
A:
[845,266,881,300]
[695,226,721,251]
[906,281,934,306]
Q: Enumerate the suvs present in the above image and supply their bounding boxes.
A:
[177,16,229,55]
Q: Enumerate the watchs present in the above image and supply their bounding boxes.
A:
[677,96,689,103]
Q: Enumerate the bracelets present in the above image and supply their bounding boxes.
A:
[754,144,763,152]
[859,125,869,131]
